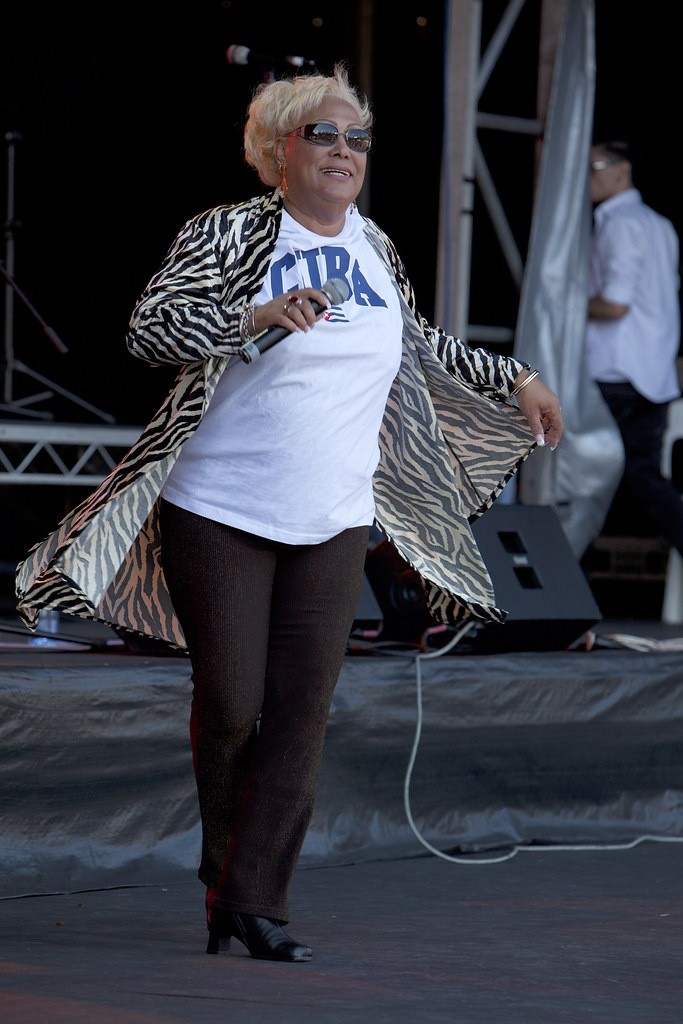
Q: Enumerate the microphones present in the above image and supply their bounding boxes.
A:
[237,279,348,363]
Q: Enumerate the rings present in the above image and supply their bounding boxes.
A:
[283,305,292,313]
[287,295,303,305]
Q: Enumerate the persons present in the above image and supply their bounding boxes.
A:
[123,62,564,961]
[583,136,682,560]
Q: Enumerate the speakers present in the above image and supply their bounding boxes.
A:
[367,503,603,655]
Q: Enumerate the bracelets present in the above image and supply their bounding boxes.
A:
[237,302,259,343]
[510,367,542,399]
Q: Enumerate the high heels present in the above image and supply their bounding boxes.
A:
[206,904,313,961]
[205,887,219,930]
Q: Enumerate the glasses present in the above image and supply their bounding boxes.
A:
[278,123,373,153]
[590,157,617,175]
